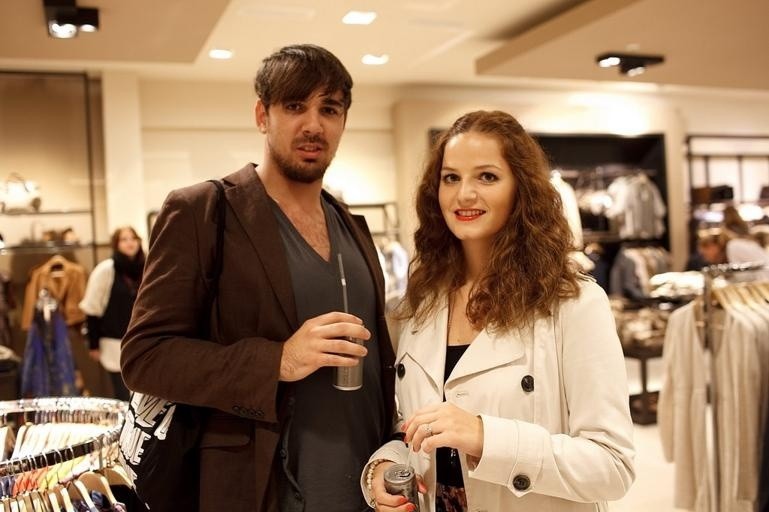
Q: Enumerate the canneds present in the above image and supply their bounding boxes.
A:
[382,462,421,512]
[333,323,365,391]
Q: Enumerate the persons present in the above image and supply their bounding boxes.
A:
[119,40,398,512]
[678,206,768,275]
[359,111,639,512]
[78,224,148,402]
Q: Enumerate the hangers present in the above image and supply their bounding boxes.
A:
[0,429,135,512]
[30,247,87,278]
[664,259,769,342]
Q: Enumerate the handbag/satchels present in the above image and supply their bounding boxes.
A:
[119,389,204,511]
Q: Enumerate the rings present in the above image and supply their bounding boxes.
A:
[424,424,434,436]
[369,497,377,509]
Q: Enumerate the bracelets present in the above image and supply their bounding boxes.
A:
[366,458,393,493]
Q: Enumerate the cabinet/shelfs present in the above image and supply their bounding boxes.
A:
[428,126,674,300]
